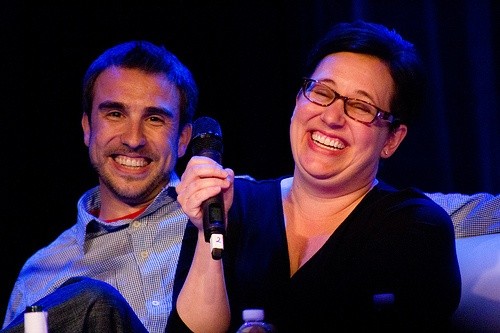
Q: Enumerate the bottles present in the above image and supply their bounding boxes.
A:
[237,309,275,333]
[25,305,42,313]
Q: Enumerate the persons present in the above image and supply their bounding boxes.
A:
[0,42,255,333]
[174,18,463,333]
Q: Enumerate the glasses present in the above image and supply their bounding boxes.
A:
[301,76,397,124]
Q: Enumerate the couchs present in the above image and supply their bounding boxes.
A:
[423,191,500,333]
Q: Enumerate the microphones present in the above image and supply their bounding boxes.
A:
[191,117,226,260]
